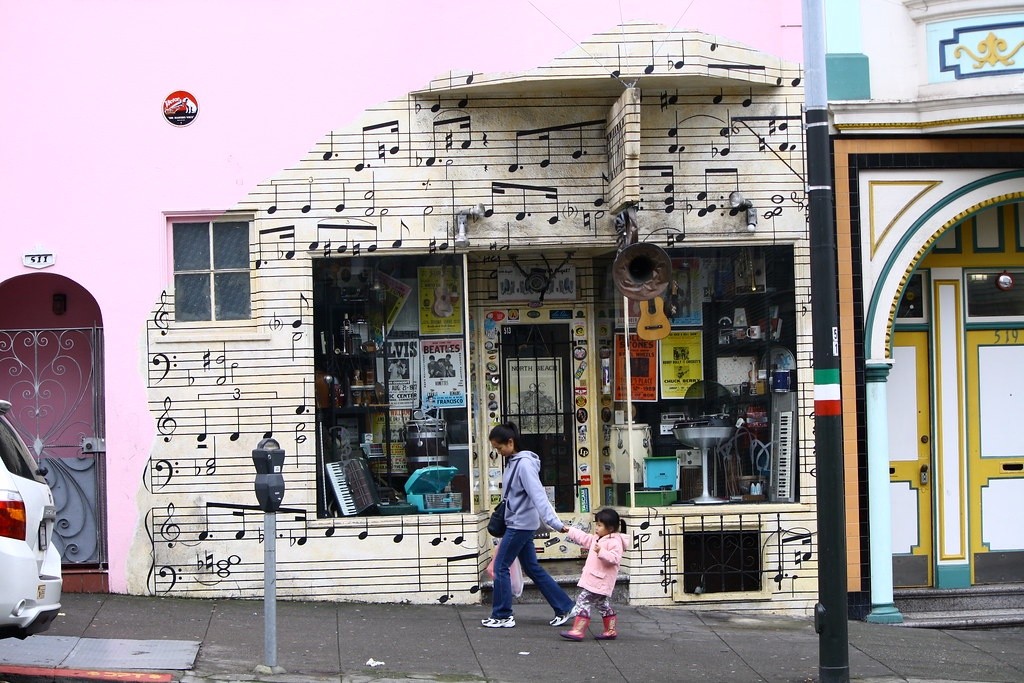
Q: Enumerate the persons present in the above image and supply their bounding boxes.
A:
[388,359,407,380]
[427,354,456,377]
[558,507,631,642]
[480,421,577,628]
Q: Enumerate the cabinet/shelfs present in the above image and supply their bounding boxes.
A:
[709,295,771,432]
[328,300,343,489]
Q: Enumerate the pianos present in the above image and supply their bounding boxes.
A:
[768,391,797,503]
[325,460,357,517]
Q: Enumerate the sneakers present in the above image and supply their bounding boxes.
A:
[481,614,515,628]
[550,604,576,626]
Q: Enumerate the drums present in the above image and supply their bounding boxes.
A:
[404,418,449,464]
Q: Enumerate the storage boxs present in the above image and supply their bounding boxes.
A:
[625,456,681,507]
[403,466,463,514]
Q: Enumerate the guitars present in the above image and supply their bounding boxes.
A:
[636,296,672,344]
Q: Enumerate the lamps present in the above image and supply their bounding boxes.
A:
[455,221,470,248]
[455,204,485,219]
[729,191,752,209]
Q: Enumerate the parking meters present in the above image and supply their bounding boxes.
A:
[252,438,286,665]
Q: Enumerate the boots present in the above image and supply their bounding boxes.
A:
[594,614,616,640]
[560,615,590,641]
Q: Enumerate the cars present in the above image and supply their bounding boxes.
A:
[0,399,64,640]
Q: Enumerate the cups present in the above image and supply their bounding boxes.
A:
[747,325,762,340]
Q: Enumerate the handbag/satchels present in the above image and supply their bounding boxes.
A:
[486,538,524,598]
[487,500,506,538]
